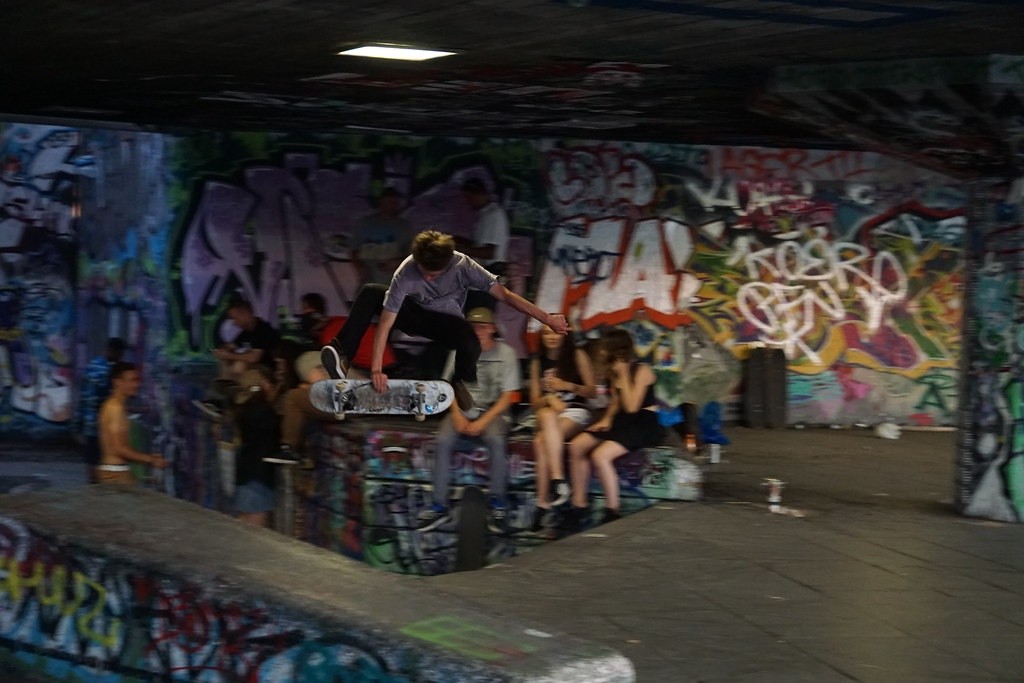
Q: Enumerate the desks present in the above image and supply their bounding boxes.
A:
[0,481,638,683]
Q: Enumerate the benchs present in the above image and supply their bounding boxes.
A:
[458,442,706,502]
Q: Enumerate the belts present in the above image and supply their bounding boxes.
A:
[98,465,131,473]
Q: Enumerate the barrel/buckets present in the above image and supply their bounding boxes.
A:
[746,346,785,428]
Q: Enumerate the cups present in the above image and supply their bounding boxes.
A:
[710,443,720,463]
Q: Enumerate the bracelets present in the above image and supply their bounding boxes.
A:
[572,383,581,393]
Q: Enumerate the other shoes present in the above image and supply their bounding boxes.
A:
[418,512,452,534]
[529,506,551,534]
[452,374,473,412]
[487,503,508,536]
[583,509,621,524]
[549,477,571,506]
[262,447,303,465]
[193,398,226,420]
[321,346,347,380]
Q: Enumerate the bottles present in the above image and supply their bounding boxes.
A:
[686,434,696,451]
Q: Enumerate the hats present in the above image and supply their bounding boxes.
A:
[468,306,502,340]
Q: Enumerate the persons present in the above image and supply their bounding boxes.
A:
[320,230,568,412]
[473,187,508,274]
[418,308,658,533]
[97,292,396,528]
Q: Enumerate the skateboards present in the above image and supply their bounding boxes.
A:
[308,378,456,422]
